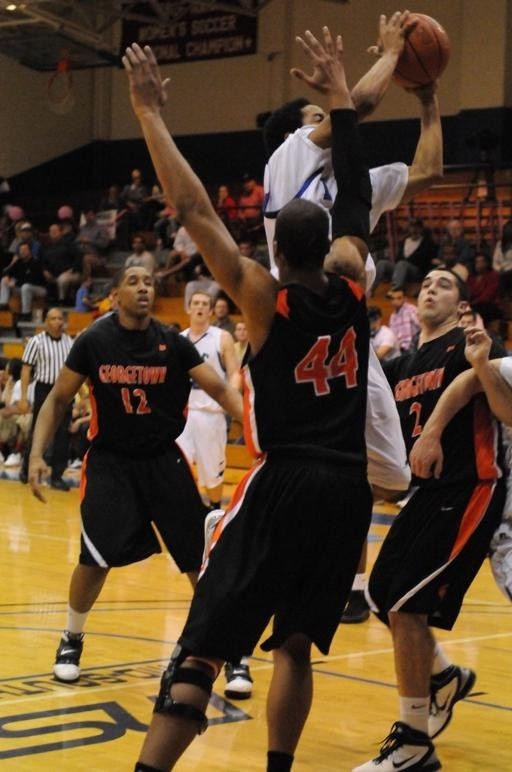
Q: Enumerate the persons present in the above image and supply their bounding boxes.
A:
[408,356,511,600]
[28,266,254,703]
[357,269,509,771]
[2,168,511,513]
[122,26,373,772]
[258,9,443,625]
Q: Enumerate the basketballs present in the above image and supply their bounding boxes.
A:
[376,12,449,88]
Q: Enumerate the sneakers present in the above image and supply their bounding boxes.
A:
[339,589,371,623]
[487,522,511,602]
[3,452,83,492]
[426,664,476,740]
[223,658,254,701]
[51,629,86,682]
[351,721,442,772]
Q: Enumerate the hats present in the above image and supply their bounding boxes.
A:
[14,220,33,232]
[407,216,424,228]
